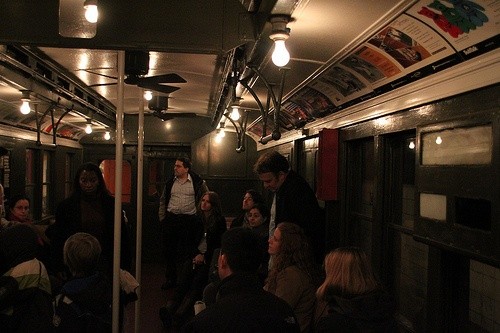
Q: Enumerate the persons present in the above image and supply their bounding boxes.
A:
[158,157,208,292]
[254,151,323,285]
[0,186,397,333]
[49,163,127,275]
